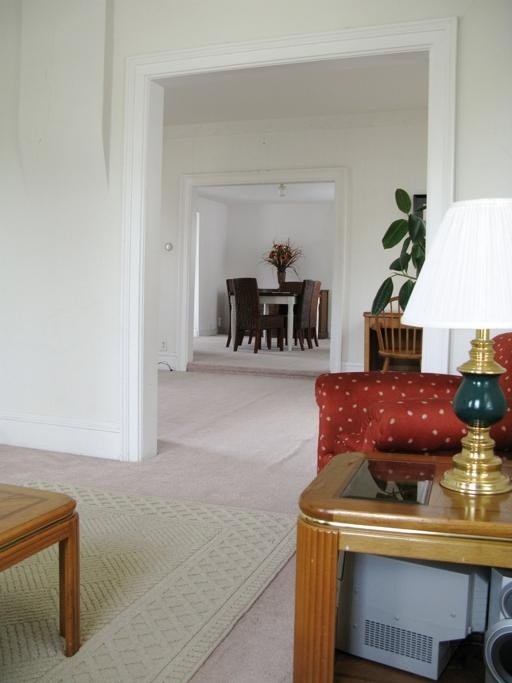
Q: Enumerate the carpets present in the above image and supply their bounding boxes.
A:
[0,483,297,683]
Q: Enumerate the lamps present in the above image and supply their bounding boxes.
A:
[278,184,287,197]
[400,199,512,496]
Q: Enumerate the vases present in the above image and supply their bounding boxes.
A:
[277,272,286,286]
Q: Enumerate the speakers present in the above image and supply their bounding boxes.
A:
[483,568,512,682]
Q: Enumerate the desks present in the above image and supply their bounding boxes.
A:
[363,312,403,372]
[0,487,81,657]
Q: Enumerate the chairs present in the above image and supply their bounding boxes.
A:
[224,278,322,354]
[315,332,512,473]
[375,295,422,371]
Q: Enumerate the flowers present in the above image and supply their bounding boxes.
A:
[259,238,305,278]
